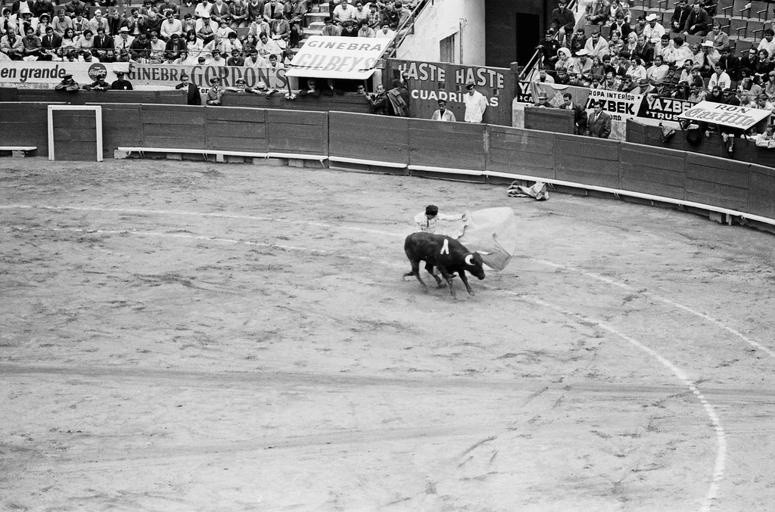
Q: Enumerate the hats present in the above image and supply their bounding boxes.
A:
[19,8,34,18]
[701,40,714,48]
[575,49,589,56]
[646,14,659,21]
[118,27,130,32]
[200,12,212,18]
[39,13,51,22]
[62,73,72,78]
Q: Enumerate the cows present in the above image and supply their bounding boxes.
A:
[402,231,486,301]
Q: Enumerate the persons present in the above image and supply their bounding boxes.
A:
[431,98,457,122]
[462,81,487,123]
[532,0,775,154]
[1,0,424,117]
[414,204,467,277]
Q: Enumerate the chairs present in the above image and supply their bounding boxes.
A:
[0,0,775,112]
